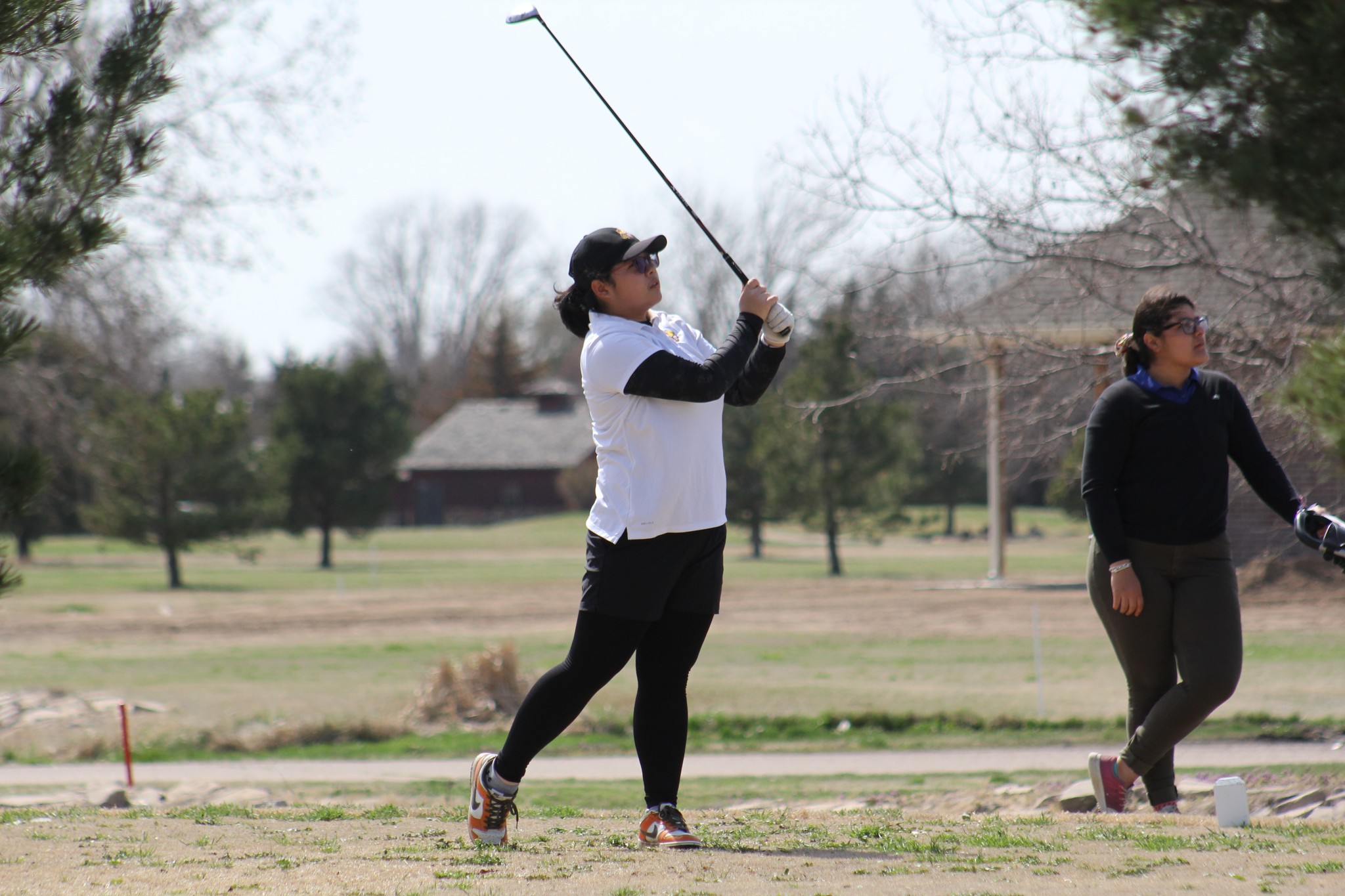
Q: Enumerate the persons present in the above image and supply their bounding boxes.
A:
[467,226,800,852]
[1080,282,1344,813]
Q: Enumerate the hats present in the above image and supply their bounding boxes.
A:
[568,228,667,283]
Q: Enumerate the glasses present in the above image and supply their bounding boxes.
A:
[607,251,660,276]
[1155,316,1209,335]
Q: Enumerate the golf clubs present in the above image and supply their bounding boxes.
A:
[502,3,792,339]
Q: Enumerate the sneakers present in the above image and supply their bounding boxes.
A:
[468,753,518,847]
[1152,801,1182,814]
[1088,752,1134,814]
[637,802,701,849]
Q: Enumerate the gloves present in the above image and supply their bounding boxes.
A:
[761,303,794,343]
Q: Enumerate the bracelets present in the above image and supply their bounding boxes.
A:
[1109,560,1134,573]
